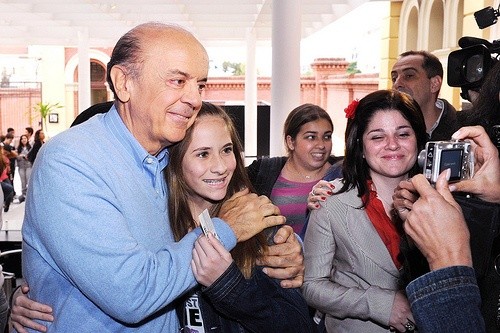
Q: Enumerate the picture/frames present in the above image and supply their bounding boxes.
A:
[49,113,58,123]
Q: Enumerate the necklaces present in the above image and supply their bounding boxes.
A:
[378,192,399,218]
[294,166,323,179]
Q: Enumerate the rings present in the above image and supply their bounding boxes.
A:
[312,190,315,196]
[404,322,413,330]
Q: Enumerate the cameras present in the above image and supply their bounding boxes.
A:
[418,139,475,188]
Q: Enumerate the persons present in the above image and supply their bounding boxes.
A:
[0,22,304,333]
[244,51,500,333]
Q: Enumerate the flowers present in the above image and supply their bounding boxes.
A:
[344,99,360,120]
